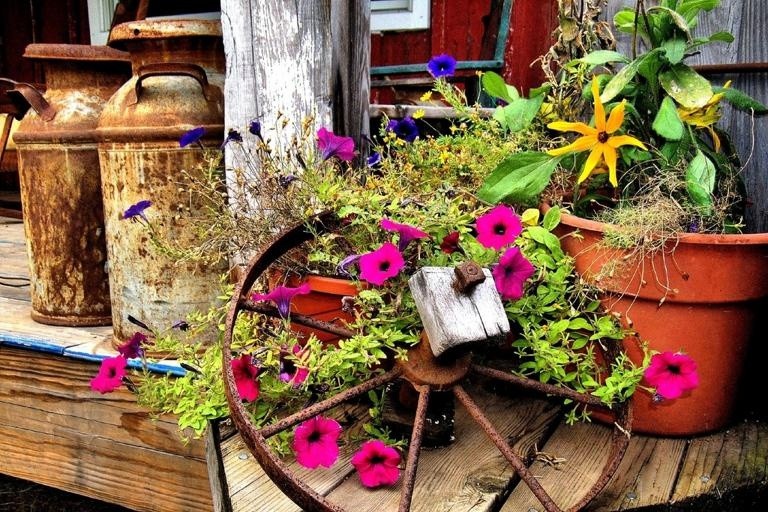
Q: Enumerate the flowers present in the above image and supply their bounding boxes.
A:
[472,0,767,210]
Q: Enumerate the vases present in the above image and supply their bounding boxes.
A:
[540,194,767,438]
